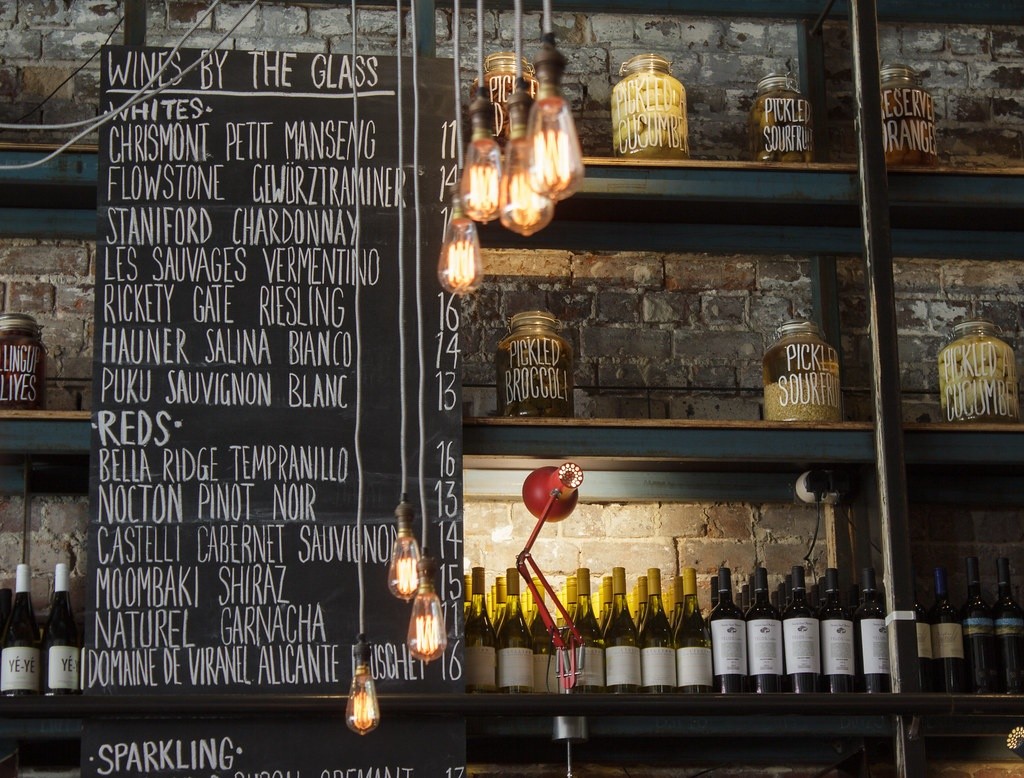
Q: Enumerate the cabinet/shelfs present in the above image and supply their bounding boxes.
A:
[1,0,1024,778]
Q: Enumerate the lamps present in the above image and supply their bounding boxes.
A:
[386,1,427,603]
[436,0,582,295]
[795,468,840,507]
[347,1,382,738]
[516,463,590,695]
[397,1,451,663]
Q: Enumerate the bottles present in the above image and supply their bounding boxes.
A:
[492,309,576,420]
[468,50,540,156]
[747,70,815,164]
[610,53,691,160]
[0,312,46,411]
[881,63,940,167]
[0,562,82,695]
[937,320,1021,424]
[761,318,843,422]
[460,556,1024,696]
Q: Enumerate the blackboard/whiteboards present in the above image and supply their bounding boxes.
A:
[80,46,472,778]
